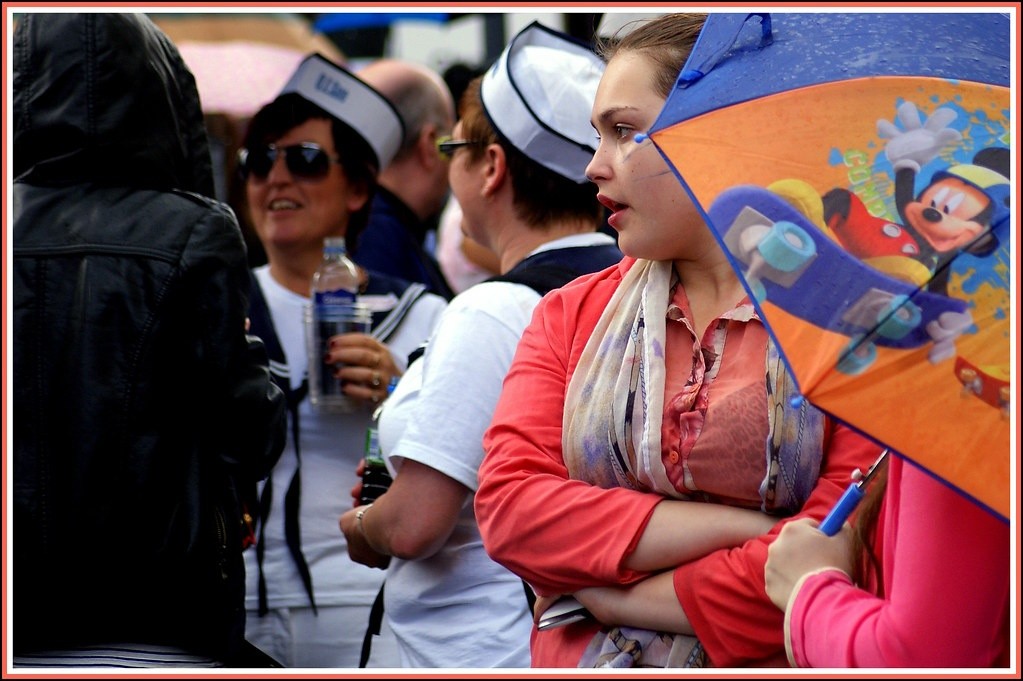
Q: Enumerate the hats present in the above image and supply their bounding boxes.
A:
[280,52,405,177]
[480,18,608,184]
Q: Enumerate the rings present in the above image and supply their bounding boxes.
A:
[368,349,381,369]
[368,370,382,388]
[369,389,379,403]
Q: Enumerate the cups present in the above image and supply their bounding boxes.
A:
[300,304,373,417]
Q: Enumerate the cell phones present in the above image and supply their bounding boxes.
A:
[536,597,588,631]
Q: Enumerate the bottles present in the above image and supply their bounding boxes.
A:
[358,376,399,504]
[311,236,360,394]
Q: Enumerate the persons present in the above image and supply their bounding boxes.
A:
[17,14,1012,670]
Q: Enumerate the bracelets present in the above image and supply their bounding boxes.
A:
[353,503,376,535]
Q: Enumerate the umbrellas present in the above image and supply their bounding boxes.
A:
[629,11,1011,537]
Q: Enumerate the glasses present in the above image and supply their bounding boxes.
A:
[239,143,343,179]
[435,135,482,158]
